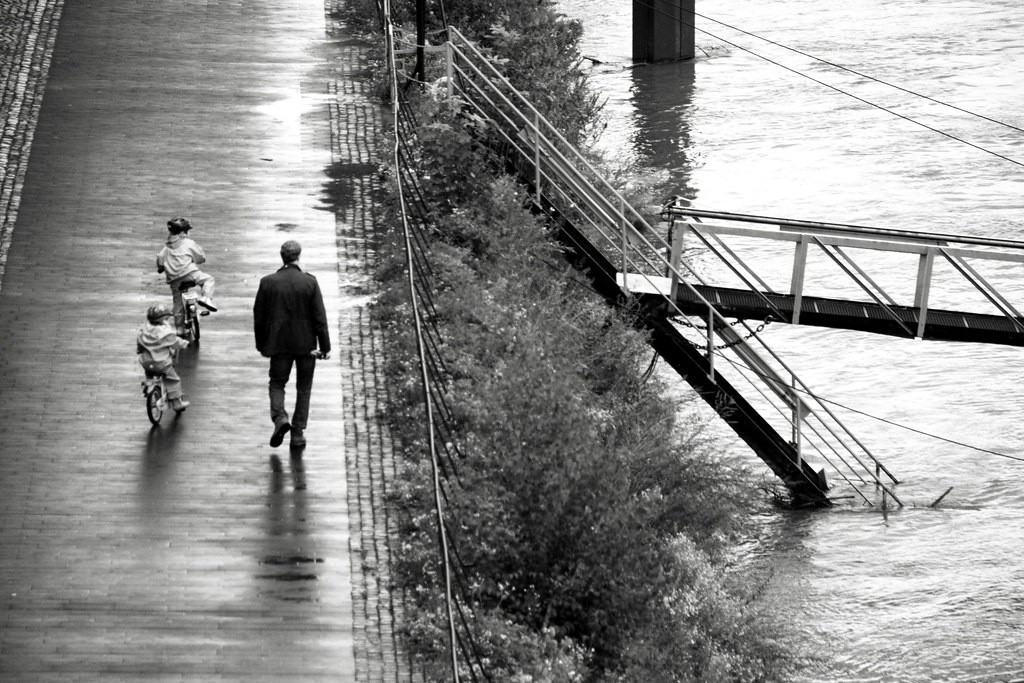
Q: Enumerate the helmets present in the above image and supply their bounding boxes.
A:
[147,303,174,320]
[166,216,193,232]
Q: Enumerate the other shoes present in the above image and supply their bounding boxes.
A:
[197,295,218,312]
[270,413,293,448]
[176,326,186,337]
[290,424,306,448]
[173,397,191,412]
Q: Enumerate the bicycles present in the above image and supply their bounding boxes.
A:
[157,265,211,348]
[136,342,194,426]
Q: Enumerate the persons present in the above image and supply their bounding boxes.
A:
[136,303,190,411]
[156,216,218,336]
[254,240,332,448]
